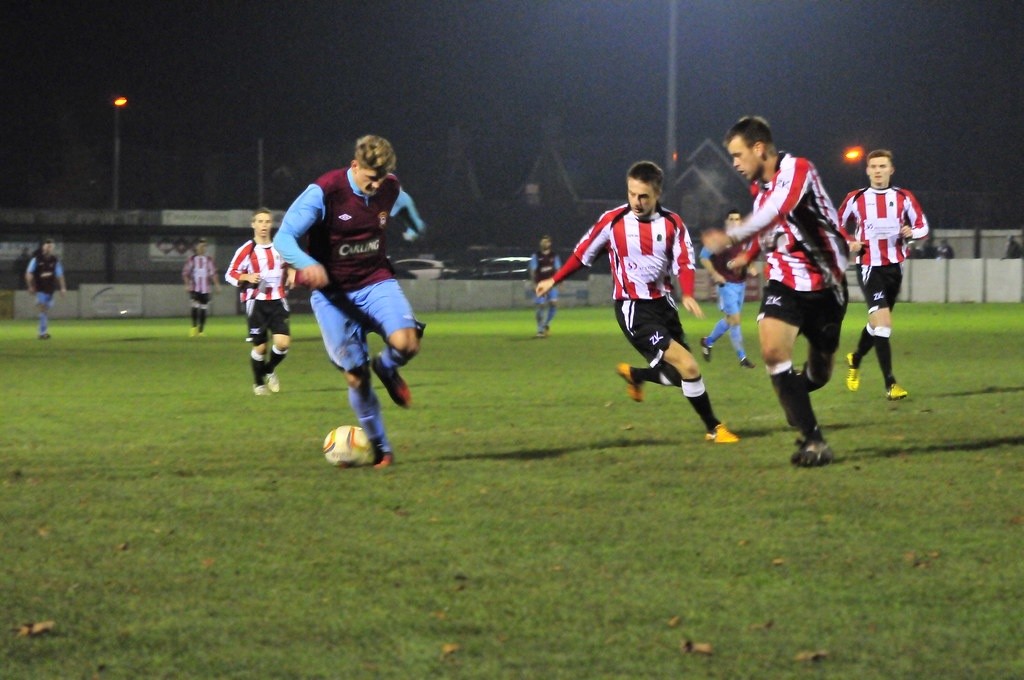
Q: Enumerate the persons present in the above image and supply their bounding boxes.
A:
[698,208,756,369]
[702,117,854,461]
[1004,234,1023,258]
[905,238,938,259]
[12,246,30,291]
[24,240,69,339]
[836,150,928,399]
[181,236,222,337]
[532,159,739,443]
[935,238,955,259]
[528,233,565,336]
[224,206,297,394]
[273,134,432,469]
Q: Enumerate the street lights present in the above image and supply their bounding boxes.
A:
[111,96,128,211]
[845,146,866,187]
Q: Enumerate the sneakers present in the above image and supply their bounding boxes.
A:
[792,438,832,465]
[700,337,713,363]
[883,384,906,401]
[705,425,739,444]
[372,353,412,408]
[739,357,755,368]
[618,362,644,402]
[369,452,394,472]
[848,351,861,390]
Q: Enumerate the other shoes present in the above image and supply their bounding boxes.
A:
[253,383,269,396]
[39,334,49,339]
[189,328,204,338]
[537,326,550,338]
[267,372,279,392]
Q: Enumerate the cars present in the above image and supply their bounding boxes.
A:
[461,255,536,282]
[387,258,458,279]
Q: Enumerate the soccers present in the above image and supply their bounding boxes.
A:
[323,426,372,466]
